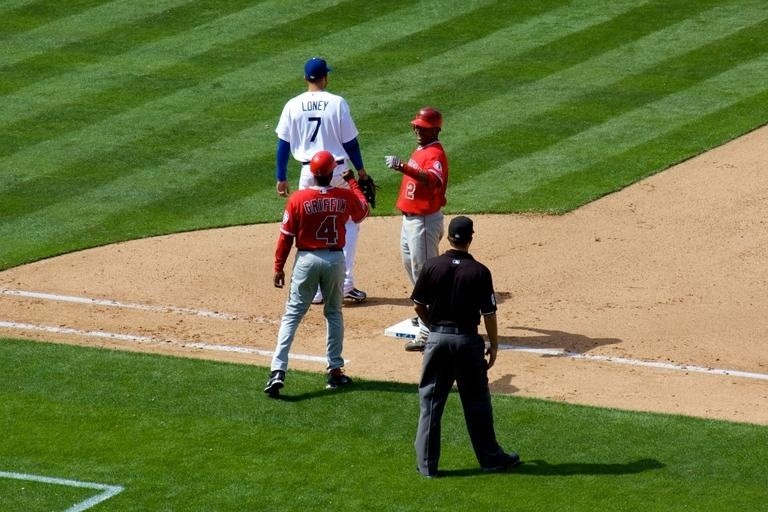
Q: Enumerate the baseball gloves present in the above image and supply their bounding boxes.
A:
[358,175,376,208]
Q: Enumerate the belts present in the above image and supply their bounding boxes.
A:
[431,326,477,335]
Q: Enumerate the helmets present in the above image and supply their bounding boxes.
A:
[311,151,338,177]
[410,108,442,136]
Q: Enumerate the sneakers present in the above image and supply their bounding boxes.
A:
[483,453,520,472]
[343,288,366,303]
[263,371,285,394]
[404,339,426,352]
[325,373,352,389]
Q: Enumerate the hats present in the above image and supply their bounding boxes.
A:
[304,58,332,81]
[448,216,474,240]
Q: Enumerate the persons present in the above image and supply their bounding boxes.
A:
[382,106,449,352]
[260,151,369,393]
[274,58,382,305]
[410,217,522,479]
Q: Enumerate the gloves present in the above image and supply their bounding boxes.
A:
[384,155,401,170]
